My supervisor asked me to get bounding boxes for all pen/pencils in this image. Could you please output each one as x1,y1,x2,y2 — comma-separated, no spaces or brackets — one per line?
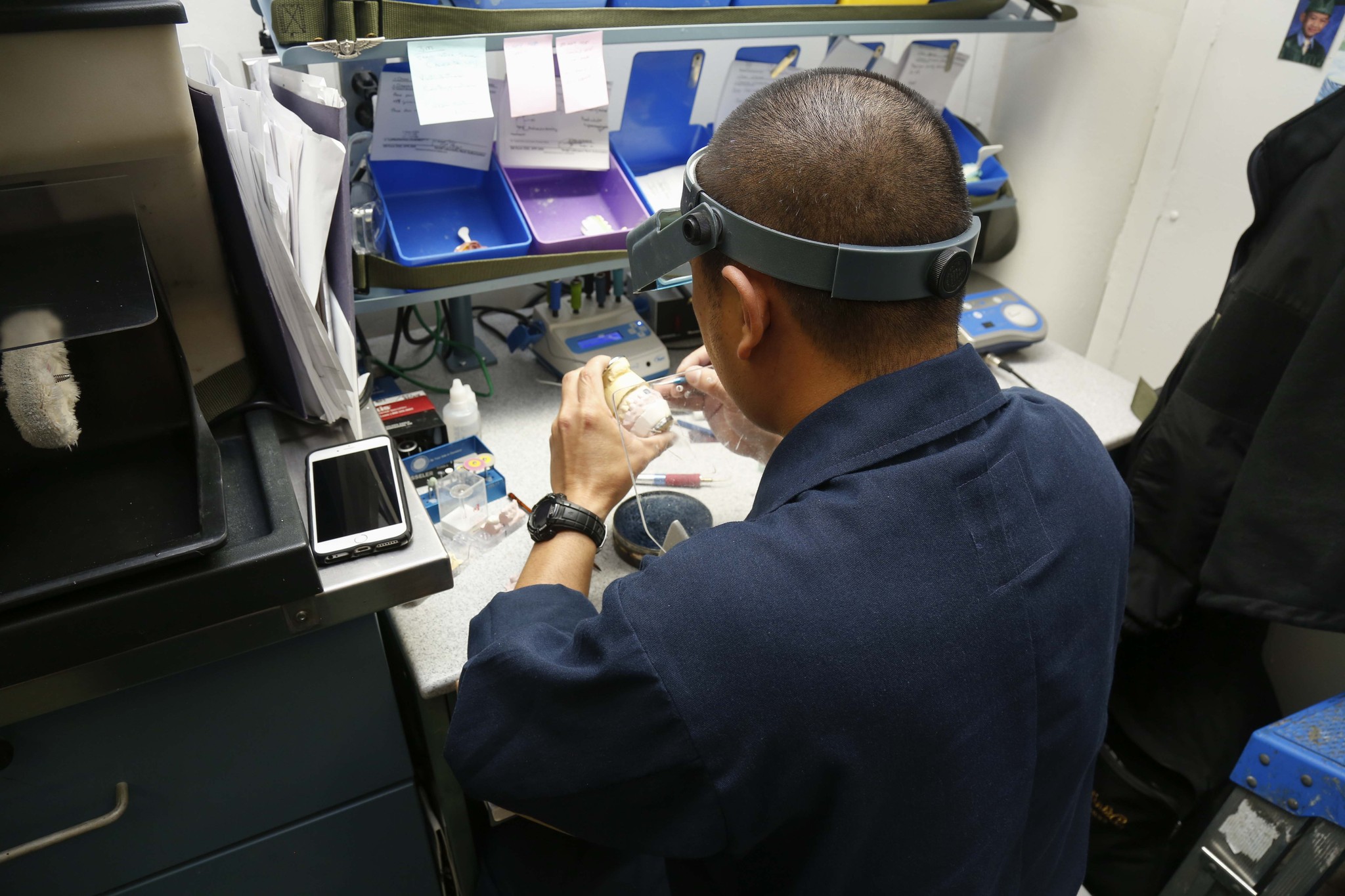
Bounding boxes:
509,494,603,573
674,417,714,437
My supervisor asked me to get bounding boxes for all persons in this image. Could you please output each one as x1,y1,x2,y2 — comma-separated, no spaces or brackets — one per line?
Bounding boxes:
1080,84,1345,896
444,66,1135,896
1279,0,1345,68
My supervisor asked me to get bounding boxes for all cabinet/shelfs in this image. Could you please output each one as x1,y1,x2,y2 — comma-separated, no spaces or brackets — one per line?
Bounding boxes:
255,0,1143,895
0,612,438,896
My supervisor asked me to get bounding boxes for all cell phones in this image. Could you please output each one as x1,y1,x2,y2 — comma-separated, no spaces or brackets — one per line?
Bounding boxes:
304,434,413,566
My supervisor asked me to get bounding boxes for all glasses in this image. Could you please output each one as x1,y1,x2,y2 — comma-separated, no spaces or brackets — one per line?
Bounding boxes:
625,203,723,296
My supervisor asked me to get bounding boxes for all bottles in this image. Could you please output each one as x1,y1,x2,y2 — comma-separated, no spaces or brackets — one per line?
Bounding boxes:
442,378,481,443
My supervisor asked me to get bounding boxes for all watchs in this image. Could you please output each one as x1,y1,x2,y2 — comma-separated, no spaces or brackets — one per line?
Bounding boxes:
527,494,607,554
